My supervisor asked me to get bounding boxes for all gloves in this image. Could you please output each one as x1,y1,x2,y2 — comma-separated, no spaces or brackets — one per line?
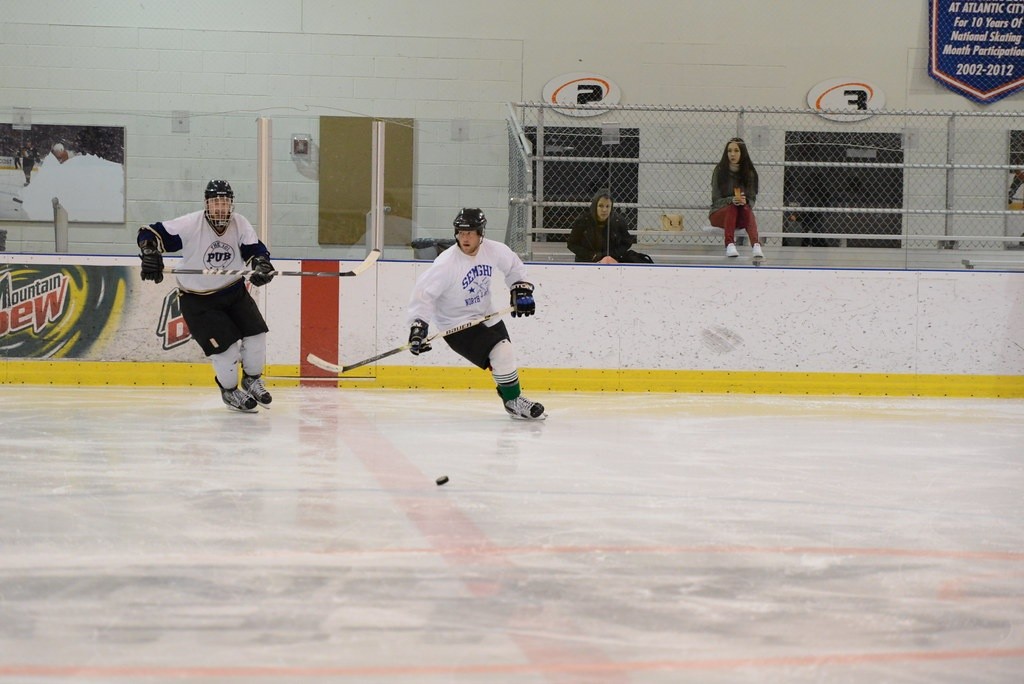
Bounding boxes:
249,256,275,287
138,246,165,284
510,281,535,318
408,318,432,355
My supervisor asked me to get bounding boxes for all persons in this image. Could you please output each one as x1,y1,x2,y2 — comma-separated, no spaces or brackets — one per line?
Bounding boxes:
708,137,764,257
408,208,548,420
9,146,21,170
23,142,43,186
136,179,275,414
51,142,68,164
567,188,632,264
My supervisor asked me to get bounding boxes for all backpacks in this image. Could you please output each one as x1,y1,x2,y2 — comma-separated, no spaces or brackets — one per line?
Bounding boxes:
620,250,654,264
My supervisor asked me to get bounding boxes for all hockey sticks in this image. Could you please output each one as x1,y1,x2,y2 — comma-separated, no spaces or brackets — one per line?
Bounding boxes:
162,248,382,277
304,306,515,374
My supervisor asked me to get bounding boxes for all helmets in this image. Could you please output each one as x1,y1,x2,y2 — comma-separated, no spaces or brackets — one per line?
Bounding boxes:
205,179,234,198
453,208,486,232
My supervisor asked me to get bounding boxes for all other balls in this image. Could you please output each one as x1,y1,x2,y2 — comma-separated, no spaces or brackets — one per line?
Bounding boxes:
435,475,450,486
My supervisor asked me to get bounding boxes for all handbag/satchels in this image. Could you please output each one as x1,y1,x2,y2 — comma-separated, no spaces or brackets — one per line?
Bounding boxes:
662,214,684,231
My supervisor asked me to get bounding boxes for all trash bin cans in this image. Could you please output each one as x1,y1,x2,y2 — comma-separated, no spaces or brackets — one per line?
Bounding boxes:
411,238,458,260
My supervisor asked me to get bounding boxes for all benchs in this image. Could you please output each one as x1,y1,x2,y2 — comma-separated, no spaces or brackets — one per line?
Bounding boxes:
530,228,1024,271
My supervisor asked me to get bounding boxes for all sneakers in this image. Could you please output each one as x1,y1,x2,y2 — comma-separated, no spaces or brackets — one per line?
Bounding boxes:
752,242,763,258
496,387,546,420
241,370,272,409
726,243,739,256
215,376,260,413
